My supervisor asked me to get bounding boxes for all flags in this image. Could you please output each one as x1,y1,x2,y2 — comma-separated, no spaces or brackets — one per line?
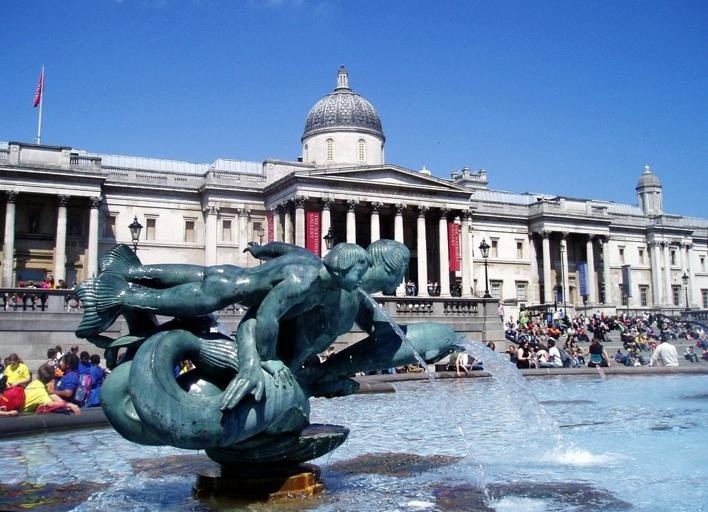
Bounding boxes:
32,68,43,109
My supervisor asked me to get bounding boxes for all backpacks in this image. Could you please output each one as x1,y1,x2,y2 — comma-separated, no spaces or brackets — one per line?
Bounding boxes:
73,374,92,402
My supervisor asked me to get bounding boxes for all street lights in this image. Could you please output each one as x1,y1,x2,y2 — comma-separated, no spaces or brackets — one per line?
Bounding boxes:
128,213,143,255
681,272,691,311
257,225,265,265
559,243,569,329
478,238,492,298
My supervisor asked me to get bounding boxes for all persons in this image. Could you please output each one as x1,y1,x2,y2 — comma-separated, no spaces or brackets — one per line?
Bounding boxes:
0,275,198,420
73,238,414,413
92,236,376,391
327,346,335,360
498,303,708,369
455,341,495,374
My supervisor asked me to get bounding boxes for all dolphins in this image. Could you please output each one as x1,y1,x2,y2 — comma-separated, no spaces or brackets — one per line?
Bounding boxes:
294,306,466,389
